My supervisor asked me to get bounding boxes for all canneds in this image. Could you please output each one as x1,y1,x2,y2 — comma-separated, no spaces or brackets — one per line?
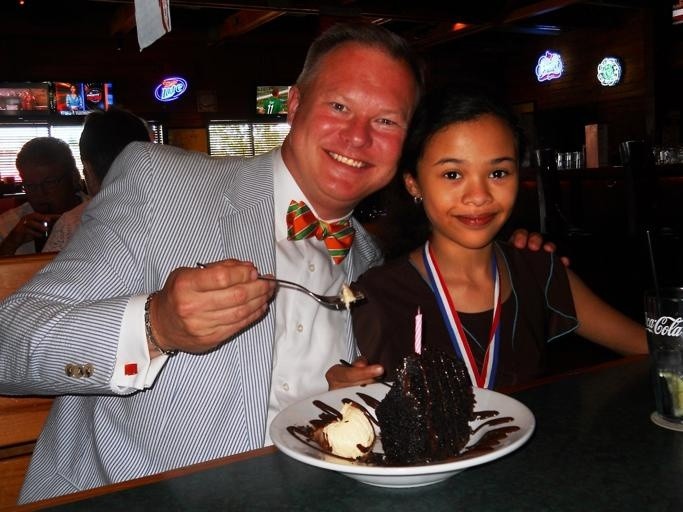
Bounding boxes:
553,151,582,172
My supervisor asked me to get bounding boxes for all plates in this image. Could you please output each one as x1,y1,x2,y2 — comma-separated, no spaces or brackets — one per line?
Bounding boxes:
265,378,536,489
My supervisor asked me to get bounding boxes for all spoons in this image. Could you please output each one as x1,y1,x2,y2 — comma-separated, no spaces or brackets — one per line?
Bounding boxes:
193,259,368,309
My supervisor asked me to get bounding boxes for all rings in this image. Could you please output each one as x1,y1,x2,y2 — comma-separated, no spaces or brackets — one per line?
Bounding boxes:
22,219,27,225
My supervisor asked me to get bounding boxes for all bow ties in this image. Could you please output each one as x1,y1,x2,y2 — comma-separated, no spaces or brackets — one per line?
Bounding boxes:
286,200,356,265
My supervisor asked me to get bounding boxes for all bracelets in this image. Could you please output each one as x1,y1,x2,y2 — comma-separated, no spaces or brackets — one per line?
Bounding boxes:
142,289,179,359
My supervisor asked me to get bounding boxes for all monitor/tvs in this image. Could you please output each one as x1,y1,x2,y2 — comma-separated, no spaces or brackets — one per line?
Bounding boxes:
53,80,115,120
256,85,293,115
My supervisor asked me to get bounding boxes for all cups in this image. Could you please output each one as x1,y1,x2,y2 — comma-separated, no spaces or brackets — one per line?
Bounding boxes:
640,286,683,423
32,204,54,254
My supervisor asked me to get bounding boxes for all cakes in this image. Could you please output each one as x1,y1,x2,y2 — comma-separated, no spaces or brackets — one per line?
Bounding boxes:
310,346,475,466
335,282,358,311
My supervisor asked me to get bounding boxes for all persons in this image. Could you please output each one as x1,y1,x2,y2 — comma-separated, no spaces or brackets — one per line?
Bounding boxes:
0,136,78,257
64,84,83,111
323,82,651,393
0,17,570,505
40,105,152,254
20,89,35,111
